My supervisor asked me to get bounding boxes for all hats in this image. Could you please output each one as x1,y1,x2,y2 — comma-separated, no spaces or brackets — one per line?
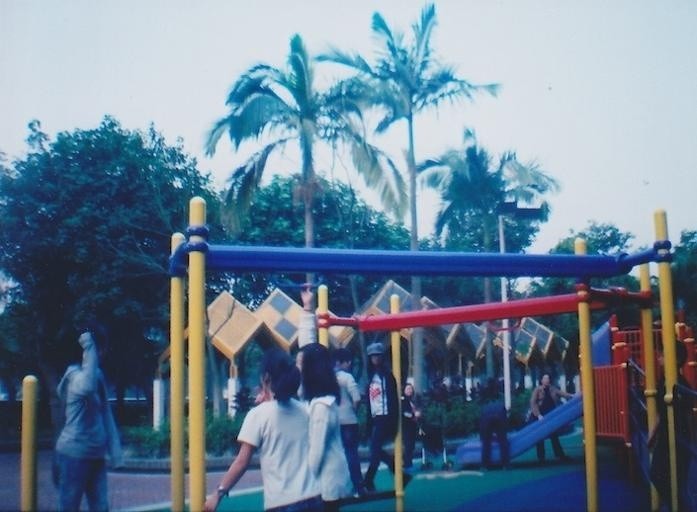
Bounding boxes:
367,342,385,356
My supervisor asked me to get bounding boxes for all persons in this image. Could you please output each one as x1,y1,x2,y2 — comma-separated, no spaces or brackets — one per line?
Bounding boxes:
478,398,517,471
643,337,692,508
201,346,326,512
253,343,354,512
364,341,413,493
47,324,127,512
528,372,575,467
398,381,424,469
334,347,369,500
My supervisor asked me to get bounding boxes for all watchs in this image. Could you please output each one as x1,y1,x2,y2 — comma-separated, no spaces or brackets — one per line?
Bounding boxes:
215,483,231,499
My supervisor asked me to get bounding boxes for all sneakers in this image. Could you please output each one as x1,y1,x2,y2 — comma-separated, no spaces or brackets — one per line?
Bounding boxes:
349,472,413,499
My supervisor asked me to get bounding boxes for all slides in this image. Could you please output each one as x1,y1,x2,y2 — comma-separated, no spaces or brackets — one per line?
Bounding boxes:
456,392,583,470
